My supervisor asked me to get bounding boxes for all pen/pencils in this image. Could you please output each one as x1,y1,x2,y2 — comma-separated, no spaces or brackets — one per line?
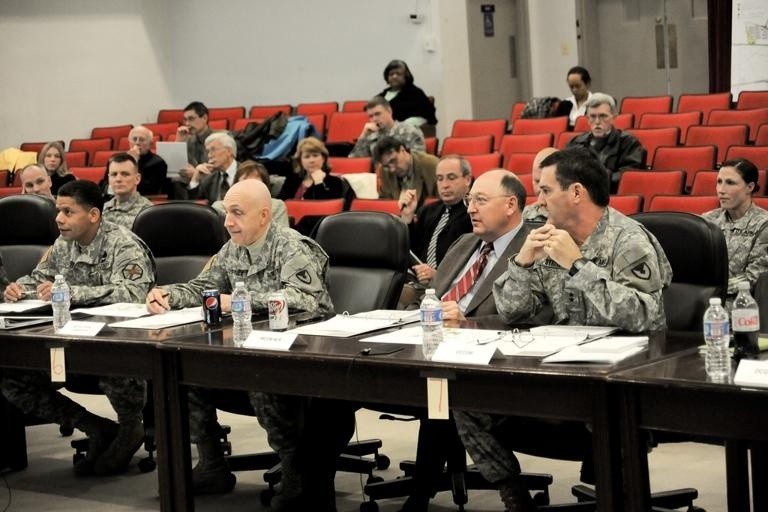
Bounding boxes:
150,293,169,303
22,291,37,295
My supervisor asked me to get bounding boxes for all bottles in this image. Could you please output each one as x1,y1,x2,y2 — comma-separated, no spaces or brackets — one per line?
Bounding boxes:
703,298,733,378
232,281,251,344
419,287,445,355
51,274,72,329
734,280,763,361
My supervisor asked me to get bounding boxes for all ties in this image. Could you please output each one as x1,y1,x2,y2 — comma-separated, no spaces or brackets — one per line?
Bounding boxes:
441,246,492,302
220,173,230,200
426,209,450,268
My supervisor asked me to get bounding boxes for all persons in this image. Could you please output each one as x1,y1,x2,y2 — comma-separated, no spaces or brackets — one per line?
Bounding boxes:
348,97,426,158
397,155,476,310
452,145,674,512
174,101,214,176
570,92,647,195
277,137,357,213
524,147,560,222
187,132,240,202
101,152,153,231
271,169,530,512
126,126,167,195
1,180,158,478
698,157,768,320
20,164,57,205
38,140,76,195
373,135,440,210
145,178,334,511
212,160,288,227
563,66,593,132
377,60,438,126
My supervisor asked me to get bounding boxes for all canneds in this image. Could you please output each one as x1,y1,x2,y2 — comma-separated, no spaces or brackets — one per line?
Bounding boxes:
267,292,290,329
201,289,223,325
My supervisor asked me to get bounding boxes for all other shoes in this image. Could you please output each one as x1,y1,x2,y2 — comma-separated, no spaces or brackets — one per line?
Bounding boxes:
271,471,304,510
191,469,235,496
499,475,534,512
278,492,337,512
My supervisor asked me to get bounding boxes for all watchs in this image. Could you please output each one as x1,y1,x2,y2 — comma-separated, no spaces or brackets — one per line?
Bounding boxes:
568,255,588,277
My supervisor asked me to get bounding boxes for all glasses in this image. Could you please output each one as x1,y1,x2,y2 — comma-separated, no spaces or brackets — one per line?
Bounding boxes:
462,192,508,208
476,328,534,348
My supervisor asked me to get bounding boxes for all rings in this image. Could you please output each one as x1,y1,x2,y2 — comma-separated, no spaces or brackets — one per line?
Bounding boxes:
422,272,425,276
546,242,549,247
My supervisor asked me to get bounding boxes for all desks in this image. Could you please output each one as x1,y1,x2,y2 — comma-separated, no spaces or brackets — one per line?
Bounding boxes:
0,298,765,510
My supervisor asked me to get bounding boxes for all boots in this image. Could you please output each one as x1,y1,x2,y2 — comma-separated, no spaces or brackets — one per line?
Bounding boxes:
74,414,120,476
94,409,146,476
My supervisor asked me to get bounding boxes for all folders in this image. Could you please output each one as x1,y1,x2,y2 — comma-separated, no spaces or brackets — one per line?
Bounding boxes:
0,315,53,329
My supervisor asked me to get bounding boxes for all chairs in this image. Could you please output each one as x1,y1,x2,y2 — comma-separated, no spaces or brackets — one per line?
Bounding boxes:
0,91,768,511
